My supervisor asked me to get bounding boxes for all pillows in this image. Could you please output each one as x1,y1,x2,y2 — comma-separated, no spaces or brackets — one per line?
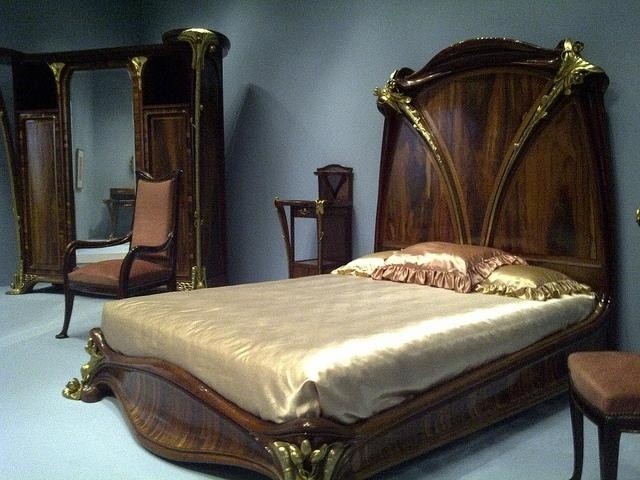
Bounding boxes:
331,241,591,301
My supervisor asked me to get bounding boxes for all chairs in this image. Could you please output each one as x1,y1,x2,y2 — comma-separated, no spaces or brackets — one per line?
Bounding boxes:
56,168,183,341
568,209,640,479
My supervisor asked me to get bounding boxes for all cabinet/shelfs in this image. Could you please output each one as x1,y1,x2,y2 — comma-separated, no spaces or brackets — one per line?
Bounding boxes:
0,27,231,294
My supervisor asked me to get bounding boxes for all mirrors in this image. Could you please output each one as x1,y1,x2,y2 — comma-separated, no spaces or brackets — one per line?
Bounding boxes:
65,68,137,269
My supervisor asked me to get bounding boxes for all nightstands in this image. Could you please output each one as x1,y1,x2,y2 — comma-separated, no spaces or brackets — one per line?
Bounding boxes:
274,165,351,278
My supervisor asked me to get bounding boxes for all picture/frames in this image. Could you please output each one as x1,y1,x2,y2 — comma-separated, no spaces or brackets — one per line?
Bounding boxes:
75,148,84,190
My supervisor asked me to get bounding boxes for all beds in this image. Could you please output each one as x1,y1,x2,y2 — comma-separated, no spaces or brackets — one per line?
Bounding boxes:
60,37,621,480
